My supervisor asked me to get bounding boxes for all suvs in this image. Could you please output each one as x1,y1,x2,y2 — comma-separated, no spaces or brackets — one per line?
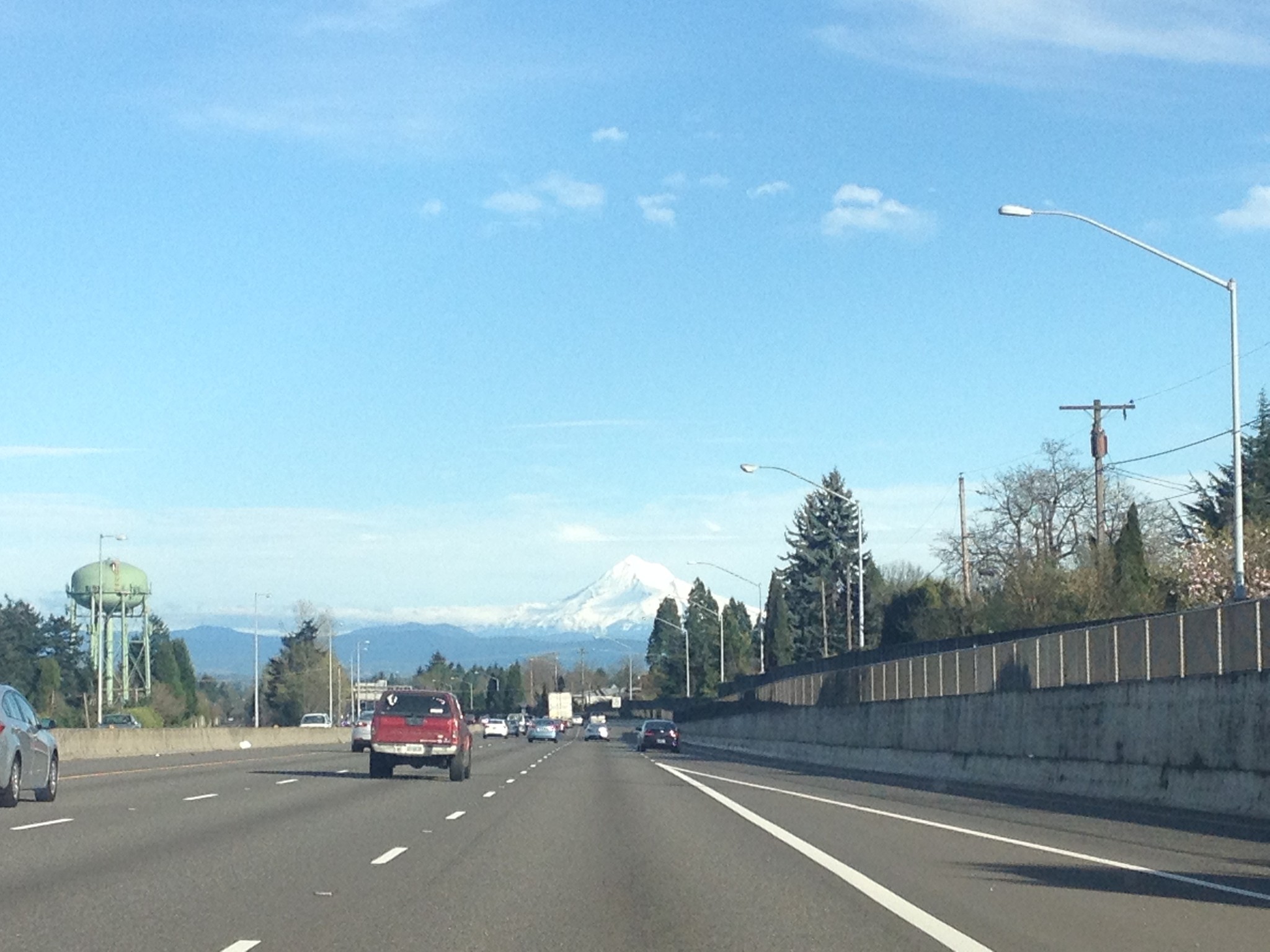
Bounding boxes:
368,687,474,782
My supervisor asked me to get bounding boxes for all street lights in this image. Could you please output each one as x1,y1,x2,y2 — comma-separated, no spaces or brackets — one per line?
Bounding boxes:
593,634,633,702
997,205,1250,603
641,616,690,698
739,463,866,648
680,597,724,683
328,621,344,726
450,676,473,710
356,638,370,726
432,679,453,693
254,590,273,729
687,560,766,675
96,531,127,726
474,670,499,691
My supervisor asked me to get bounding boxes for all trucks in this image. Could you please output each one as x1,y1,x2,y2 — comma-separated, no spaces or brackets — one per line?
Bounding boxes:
547,691,573,722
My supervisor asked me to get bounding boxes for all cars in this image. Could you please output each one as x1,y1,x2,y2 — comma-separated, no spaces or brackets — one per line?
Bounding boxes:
462,708,611,743
350,709,375,753
100,712,142,729
583,723,611,742
0,683,60,809
298,712,332,729
635,718,680,753
